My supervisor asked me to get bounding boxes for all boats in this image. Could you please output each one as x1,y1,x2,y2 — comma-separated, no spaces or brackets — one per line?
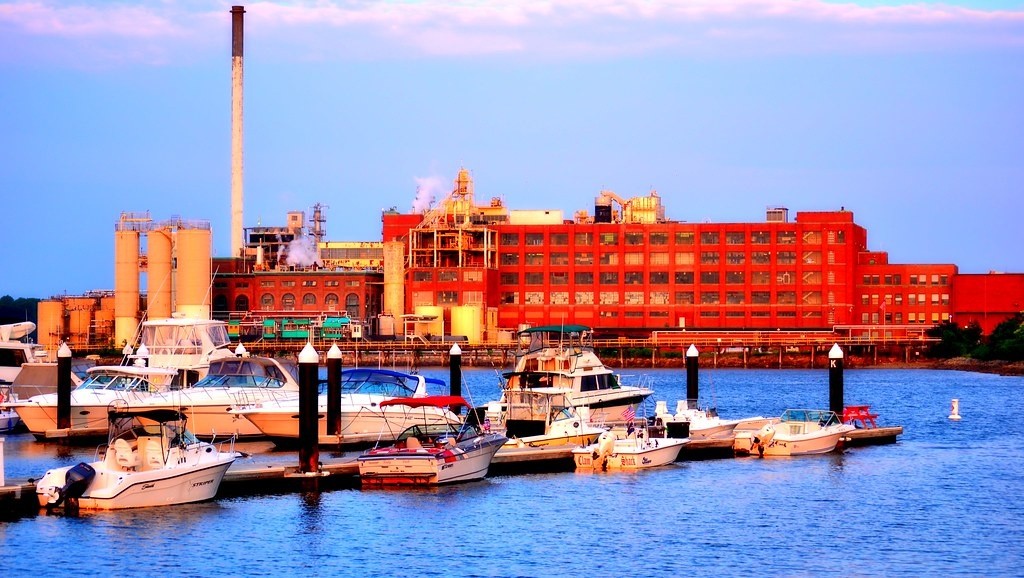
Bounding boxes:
732,409,857,456
356,397,509,487
674,399,763,430
0,366,179,441
502,406,605,449
571,417,691,469
481,325,654,437
228,367,460,448
35,409,243,510
114,353,299,439
0,385,20,432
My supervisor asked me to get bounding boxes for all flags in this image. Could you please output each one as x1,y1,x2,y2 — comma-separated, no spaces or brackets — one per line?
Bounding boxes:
621,405,635,422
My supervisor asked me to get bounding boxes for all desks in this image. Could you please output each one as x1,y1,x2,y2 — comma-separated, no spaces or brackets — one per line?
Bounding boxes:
843,406,877,430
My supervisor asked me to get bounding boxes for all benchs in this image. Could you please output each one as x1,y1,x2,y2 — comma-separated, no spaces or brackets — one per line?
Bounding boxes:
842,414,880,430
